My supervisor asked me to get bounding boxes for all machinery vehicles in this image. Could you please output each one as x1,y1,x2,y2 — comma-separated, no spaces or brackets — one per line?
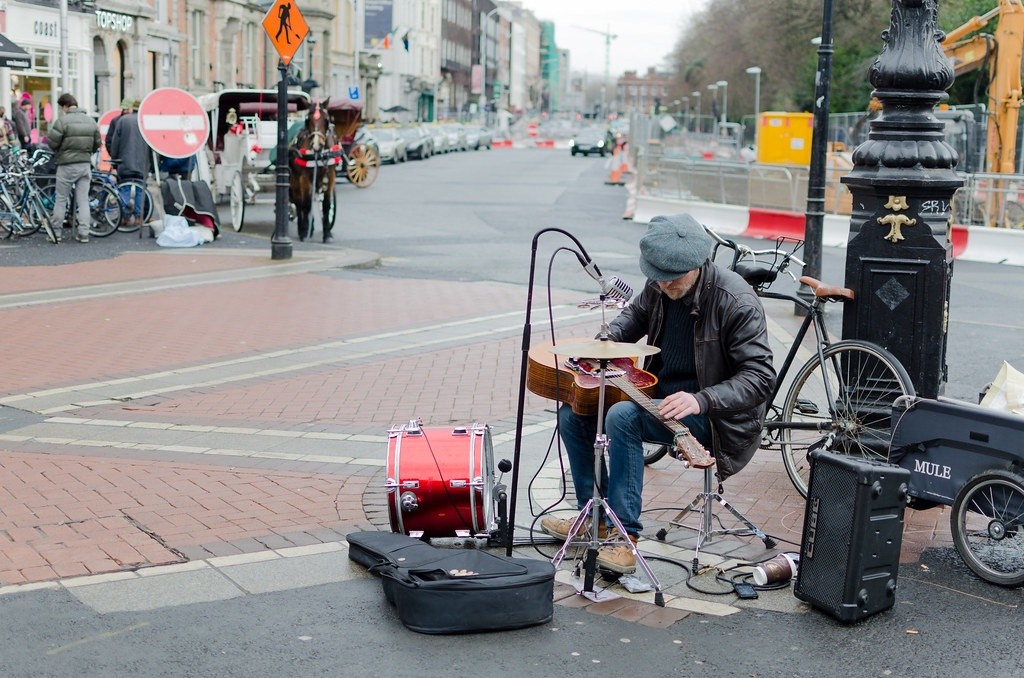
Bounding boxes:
749,1,1024,230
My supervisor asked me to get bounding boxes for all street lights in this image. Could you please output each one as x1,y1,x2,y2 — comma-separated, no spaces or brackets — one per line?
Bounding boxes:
706,84,720,122
674,100,683,126
717,81,729,122
681,96,690,129
745,65,762,147
691,91,702,133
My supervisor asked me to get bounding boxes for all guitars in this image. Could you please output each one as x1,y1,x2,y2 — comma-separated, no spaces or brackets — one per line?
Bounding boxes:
527,335,716,470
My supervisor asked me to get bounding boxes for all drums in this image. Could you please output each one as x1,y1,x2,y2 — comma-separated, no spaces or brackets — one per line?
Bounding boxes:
386,424,496,540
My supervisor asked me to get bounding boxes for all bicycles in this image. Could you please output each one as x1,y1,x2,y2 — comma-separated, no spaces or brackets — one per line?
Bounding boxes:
0,142,154,244
699,221,917,502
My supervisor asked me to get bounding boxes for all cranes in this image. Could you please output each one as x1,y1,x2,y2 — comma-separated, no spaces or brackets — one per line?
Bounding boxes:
572,23,618,76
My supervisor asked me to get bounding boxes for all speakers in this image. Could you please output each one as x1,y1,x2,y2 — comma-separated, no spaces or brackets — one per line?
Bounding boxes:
793,451,909,626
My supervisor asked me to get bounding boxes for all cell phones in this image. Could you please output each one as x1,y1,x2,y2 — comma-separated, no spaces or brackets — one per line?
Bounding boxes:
732,583,758,598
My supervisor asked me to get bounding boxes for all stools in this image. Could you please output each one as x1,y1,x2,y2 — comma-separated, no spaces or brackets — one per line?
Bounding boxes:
654,448,776,576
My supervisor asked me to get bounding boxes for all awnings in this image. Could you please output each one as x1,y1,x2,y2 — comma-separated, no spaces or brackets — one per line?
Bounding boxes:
0,34,31,68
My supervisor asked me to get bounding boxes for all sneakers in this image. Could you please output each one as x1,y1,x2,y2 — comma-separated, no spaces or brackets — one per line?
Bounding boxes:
595,527,637,574
541,514,607,542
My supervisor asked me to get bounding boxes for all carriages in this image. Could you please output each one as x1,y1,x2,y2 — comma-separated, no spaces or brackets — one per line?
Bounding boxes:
190,86,380,243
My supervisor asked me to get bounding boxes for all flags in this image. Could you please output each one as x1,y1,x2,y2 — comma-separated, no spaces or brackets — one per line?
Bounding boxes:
401,34,408,51
384,31,394,49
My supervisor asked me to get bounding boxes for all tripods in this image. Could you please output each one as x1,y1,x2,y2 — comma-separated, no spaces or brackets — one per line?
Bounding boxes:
551,360,666,608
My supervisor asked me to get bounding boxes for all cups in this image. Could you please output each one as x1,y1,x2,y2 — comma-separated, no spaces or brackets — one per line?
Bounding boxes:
753,553,796,586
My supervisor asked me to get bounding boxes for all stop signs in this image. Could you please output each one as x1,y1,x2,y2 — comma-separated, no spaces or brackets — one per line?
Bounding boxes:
96,109,124,153
526,124,541,138
137,87,211,159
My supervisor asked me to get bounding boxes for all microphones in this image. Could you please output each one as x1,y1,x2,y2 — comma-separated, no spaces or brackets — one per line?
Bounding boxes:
576,254,635,303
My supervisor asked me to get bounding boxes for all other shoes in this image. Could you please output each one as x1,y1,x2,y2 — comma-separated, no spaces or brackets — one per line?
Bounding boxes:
76,234,88,243
47,235,61,241
121,217,130,226
134,216,141,225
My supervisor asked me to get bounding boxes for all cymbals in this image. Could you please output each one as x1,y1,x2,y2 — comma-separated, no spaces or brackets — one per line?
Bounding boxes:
548,339,663,359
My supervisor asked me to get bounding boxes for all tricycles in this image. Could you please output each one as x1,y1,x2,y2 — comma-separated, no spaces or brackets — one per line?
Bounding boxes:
885,392,1024,589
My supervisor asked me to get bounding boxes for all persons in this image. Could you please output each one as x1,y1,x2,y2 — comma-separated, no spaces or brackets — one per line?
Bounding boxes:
541,214,777,573
111,98,150,225
15,100,31,154
46,94,102,243
159,155,196,181
0,106,15,173
104,98,134,170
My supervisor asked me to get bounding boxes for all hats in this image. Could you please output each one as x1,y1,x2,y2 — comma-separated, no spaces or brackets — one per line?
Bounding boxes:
132,99,142,111
639,212,712,282
120,98,134,110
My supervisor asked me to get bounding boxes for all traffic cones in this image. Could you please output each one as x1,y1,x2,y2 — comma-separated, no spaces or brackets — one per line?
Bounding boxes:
502,130,513,148
489,129,502,150
613,144,633,174
604,147,626,186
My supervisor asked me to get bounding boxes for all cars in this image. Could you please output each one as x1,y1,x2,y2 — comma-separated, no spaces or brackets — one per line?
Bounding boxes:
397,125,433,161
570,125,615,157
422,121,493,157
370,127,408,164
